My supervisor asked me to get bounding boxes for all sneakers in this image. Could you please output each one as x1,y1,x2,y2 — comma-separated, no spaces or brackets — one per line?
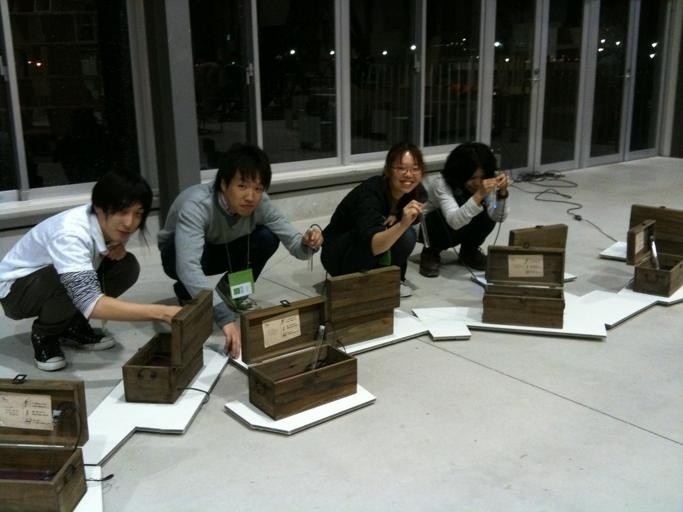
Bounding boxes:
29,318,66,371
399,281,412,298
62,321,115,351
174,282,195,306
216,286,262,313
419,244,440,277
457,245,488,270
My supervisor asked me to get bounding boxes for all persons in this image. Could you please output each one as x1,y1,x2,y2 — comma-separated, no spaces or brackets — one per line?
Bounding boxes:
319,140,430,298
411,141,510,278
156,143,324,358
1,170,184,372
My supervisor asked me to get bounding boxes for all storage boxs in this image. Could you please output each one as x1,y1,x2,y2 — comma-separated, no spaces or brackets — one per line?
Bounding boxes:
240,295,357,421
509,224,568,248
481,245,565,329
121,290,214,404
0,374,89,512
630,204,683,255
325,265,401,349
627,219,683,297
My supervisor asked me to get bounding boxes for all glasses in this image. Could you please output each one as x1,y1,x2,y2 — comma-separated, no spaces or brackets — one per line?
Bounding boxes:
388,165,422,176
